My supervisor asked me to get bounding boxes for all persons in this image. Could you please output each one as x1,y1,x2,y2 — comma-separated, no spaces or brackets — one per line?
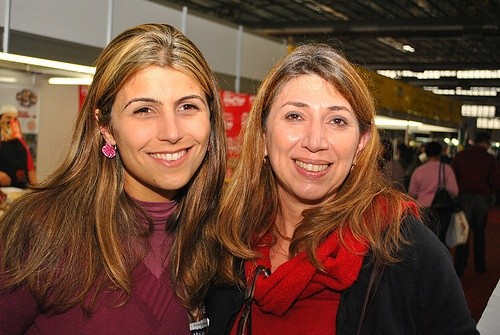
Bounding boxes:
453,133,498,275
206,44,480,335
380,139,500,209
409,141,458,243
0,22,232,335
0,107,36,188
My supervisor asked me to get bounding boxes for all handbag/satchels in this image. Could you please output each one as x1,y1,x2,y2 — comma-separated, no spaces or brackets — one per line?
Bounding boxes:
423,187,469,246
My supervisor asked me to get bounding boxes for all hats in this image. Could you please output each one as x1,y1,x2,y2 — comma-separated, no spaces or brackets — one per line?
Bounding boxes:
0,104,18,116
